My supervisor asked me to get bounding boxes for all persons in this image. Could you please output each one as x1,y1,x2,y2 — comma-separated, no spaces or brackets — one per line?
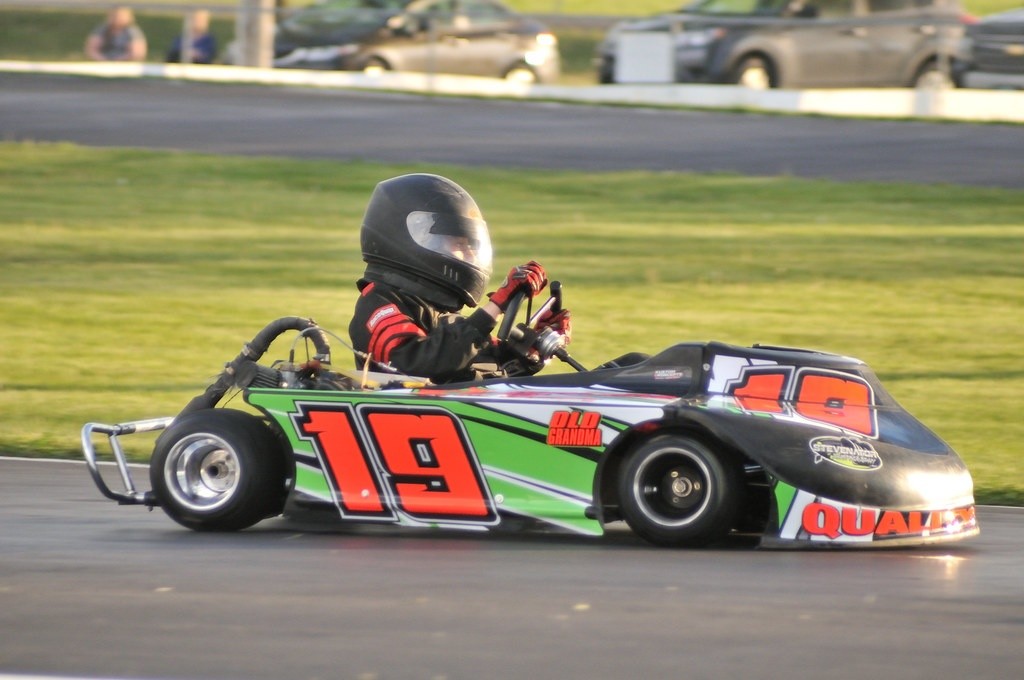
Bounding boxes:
77,2,151,65
347,174,571,383
166,0,223,65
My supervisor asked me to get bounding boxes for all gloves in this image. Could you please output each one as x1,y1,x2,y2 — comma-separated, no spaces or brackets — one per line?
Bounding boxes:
528,309,571,357
487,260,548,313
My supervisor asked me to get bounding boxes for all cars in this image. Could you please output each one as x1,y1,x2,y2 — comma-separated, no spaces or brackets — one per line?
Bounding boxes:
226,0,560,83
599,0,972,92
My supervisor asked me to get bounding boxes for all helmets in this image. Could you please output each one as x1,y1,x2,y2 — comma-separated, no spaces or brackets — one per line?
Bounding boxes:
360,173,494,312
